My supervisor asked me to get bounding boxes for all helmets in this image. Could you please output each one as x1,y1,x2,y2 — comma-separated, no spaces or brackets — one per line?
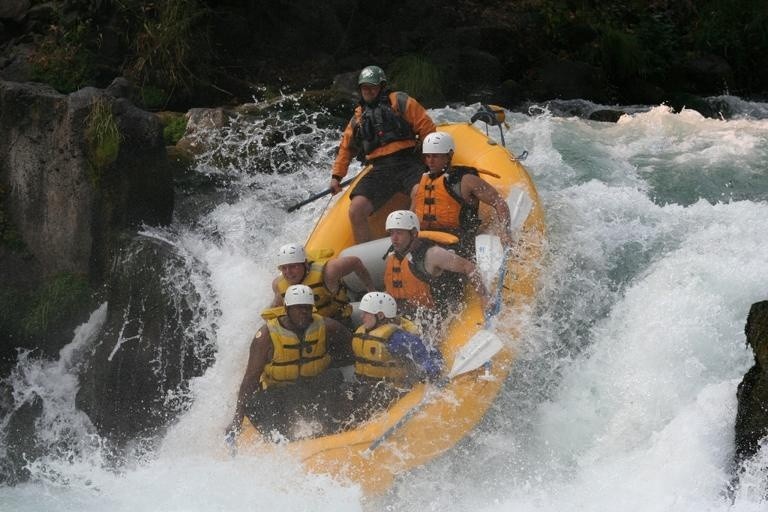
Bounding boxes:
358,65,387,87
359,291,397,319
422,131,455,155
277,242,306,267
283,284,315,308
385,209,421,234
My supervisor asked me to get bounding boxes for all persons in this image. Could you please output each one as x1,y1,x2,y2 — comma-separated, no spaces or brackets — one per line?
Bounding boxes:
268,240,377,329
221,285,350,459
379,206,492,340
409,130,511,254
329,63,436,247
347,289,442,423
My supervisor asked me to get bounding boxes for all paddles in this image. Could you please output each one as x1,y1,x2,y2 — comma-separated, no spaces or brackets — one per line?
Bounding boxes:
474,234,503,382
490,184,535,317
360,329,505,459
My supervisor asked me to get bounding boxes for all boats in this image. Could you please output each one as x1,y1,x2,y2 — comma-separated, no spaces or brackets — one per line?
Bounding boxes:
221,91,545,510
